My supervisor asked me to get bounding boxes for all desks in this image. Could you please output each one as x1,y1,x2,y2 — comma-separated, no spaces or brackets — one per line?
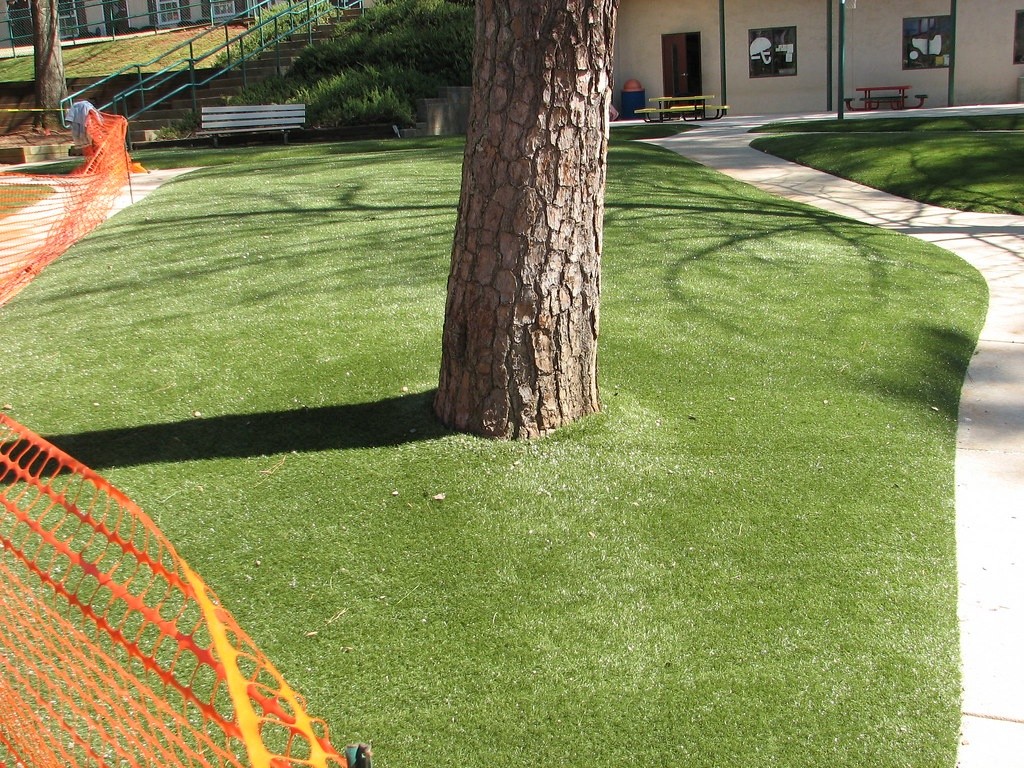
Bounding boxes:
855,85,912,111
648,95,719,123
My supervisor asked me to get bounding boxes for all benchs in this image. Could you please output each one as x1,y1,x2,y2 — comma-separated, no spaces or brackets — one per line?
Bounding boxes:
843,97,854,111
197,103,309,148
910,94,929,108
634,104,729,123
858,95,909,111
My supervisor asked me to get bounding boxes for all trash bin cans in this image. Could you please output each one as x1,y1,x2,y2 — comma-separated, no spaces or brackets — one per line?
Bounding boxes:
620,79,645,120
